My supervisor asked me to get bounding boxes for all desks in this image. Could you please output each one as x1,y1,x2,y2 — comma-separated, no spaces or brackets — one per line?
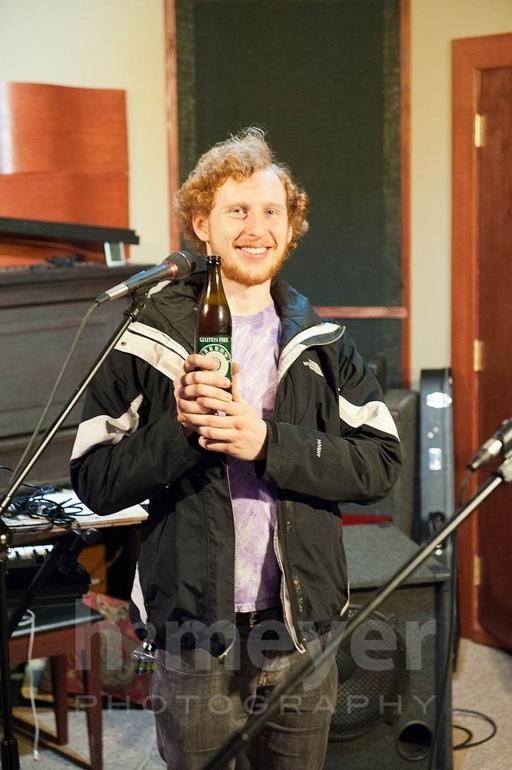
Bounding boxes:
1,487,148,770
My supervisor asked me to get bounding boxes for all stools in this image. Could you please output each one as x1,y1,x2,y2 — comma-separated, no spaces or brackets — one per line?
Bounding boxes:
1,601,109,769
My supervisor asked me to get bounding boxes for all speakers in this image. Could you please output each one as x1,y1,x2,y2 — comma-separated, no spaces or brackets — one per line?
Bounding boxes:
256,524,453,770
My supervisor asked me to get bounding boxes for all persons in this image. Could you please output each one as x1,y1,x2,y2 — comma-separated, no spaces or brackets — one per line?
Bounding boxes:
70,127,402,770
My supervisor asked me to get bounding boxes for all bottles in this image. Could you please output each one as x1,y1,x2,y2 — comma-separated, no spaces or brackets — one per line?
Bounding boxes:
194,254,234,416
130,641,153,672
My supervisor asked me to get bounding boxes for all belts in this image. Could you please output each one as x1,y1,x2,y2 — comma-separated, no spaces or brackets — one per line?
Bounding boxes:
235,604,285,631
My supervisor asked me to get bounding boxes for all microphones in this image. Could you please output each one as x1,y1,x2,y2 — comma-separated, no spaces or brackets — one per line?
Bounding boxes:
467,418,512,470
96,251,192,307
